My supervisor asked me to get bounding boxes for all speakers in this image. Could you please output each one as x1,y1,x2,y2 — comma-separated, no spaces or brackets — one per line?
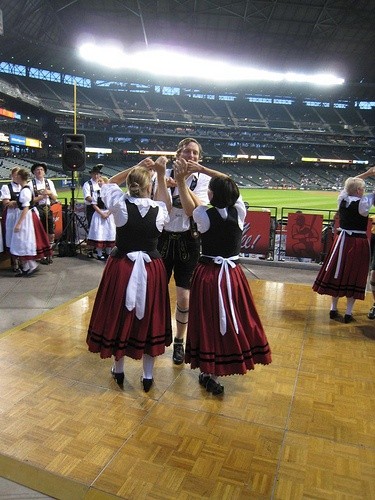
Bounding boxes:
62,134,86,172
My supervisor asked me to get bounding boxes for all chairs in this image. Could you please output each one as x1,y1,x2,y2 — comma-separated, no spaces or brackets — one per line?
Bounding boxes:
0,158,66,180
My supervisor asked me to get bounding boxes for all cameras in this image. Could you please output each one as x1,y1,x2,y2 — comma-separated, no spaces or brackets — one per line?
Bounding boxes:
173,195,182,208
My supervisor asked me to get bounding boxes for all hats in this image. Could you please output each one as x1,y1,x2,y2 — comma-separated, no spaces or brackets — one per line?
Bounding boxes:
31,163,47,174
89,166,103,174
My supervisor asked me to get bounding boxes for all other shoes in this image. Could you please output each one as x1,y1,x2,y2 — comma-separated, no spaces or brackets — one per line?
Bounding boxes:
26,266,40,276
87,250,92,258
15,268,30,277
40,258,48,265
46,258,52,263
12,267,21,272
98,253,110,260
368,303,375,319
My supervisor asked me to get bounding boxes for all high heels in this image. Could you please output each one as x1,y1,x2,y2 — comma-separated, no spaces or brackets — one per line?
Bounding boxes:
207,380,224,394
143,376,154,392
330,310,338,319
344,314,352,323
111,366,125,385
199,373,210,387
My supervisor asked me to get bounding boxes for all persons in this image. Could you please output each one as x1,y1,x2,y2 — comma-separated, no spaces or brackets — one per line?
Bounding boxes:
82,164,105,254
28,163,58,265
10,168,39,275
0,166,24,271
238,202,249,257
150,135,213,364
295,211,314,262
86,158,173,393
299,163,375,190
312,167,375,323
260,172,286,183
172,157,272,394
87,175,116,259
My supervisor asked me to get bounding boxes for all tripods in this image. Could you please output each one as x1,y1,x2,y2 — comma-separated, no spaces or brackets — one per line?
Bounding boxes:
52,171,88,256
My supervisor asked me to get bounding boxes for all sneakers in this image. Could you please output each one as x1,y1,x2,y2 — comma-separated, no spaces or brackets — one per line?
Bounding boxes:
173,343,185,364
